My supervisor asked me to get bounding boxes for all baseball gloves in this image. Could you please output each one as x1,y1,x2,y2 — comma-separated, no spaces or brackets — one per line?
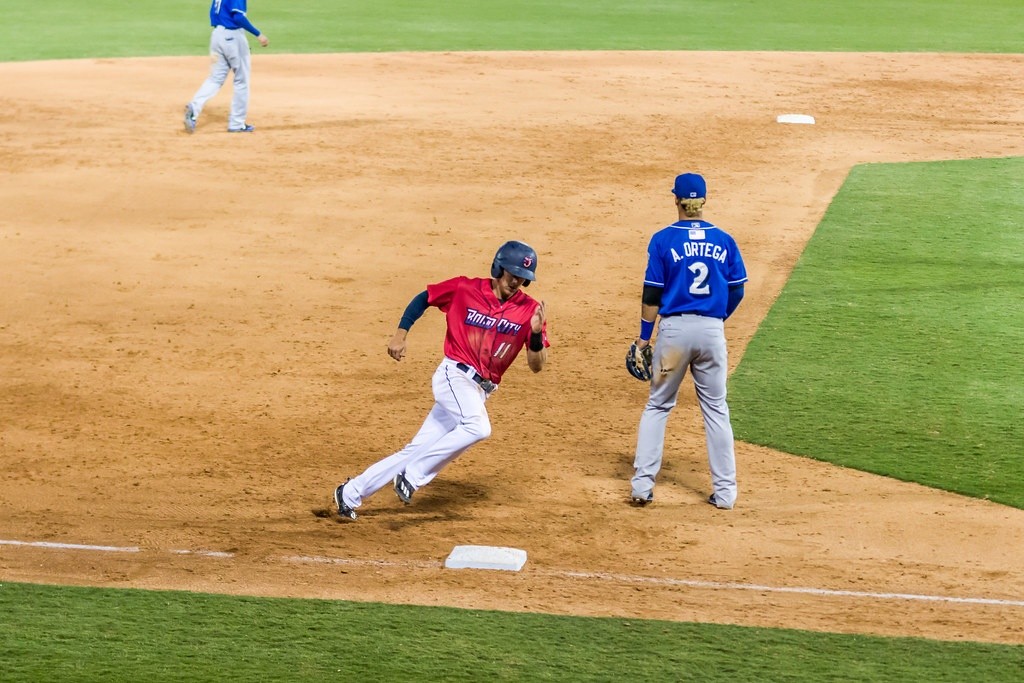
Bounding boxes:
625,342,653,382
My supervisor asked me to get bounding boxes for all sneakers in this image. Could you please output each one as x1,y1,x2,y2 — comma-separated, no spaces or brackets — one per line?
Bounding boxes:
708,493,717,505
183,103,196,132
393,470,415,505
334,477,356,520
228,124,254,132
632,490,653,503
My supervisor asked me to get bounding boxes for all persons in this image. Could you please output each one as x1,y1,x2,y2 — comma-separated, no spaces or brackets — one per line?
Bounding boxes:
333,240,550,520
626,172,748,511
182,0,269,133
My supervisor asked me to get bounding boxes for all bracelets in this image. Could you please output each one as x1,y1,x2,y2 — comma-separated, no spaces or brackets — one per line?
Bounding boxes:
529,329,543,352
640,317,656,341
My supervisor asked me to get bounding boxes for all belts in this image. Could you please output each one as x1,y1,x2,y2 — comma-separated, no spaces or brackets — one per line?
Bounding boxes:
457,363,494,394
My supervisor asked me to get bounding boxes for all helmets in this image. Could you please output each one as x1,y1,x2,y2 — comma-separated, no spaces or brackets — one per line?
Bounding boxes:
491,241,537,287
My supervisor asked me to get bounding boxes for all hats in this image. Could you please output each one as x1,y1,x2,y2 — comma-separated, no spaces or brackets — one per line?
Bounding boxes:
671,173,706,199
